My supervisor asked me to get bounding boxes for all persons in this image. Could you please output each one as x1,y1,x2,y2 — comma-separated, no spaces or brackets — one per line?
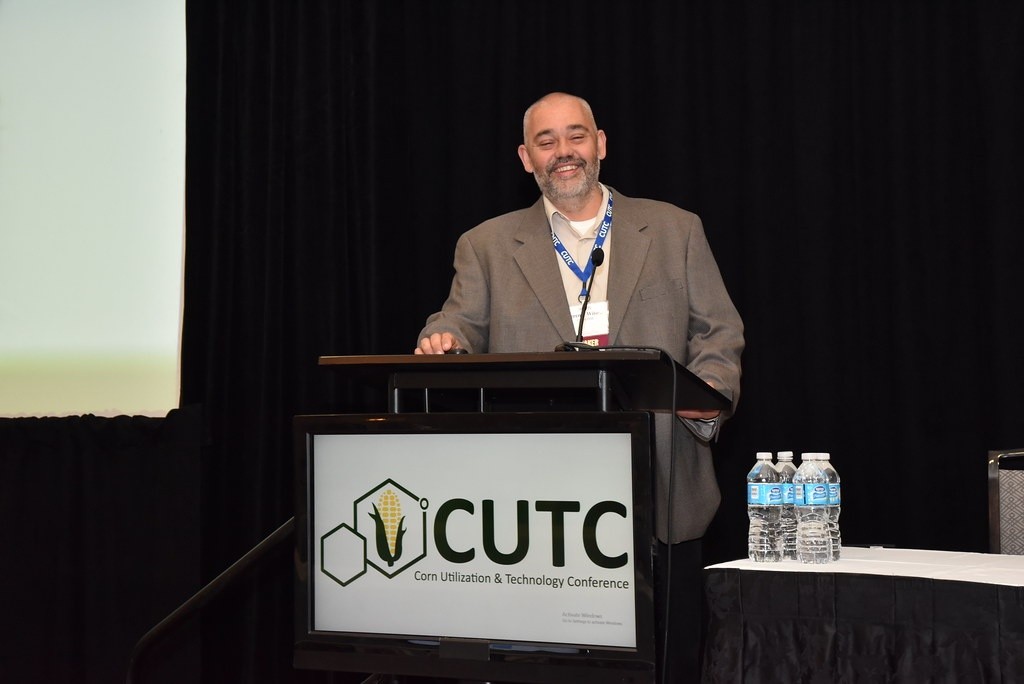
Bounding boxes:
414,92,746,684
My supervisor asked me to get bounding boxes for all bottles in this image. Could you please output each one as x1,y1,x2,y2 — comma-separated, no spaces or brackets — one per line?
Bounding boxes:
793,453,834,565
746,451,781,563
816,454,841,562
773,451,801,561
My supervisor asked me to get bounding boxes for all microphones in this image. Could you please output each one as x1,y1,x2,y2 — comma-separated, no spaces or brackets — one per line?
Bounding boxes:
555,248,604,353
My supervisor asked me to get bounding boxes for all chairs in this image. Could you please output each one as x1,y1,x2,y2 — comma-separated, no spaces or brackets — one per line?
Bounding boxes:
988,448,1024,555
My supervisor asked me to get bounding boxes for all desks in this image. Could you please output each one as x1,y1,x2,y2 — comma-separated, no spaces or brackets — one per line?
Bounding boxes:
702,546,1024,684
